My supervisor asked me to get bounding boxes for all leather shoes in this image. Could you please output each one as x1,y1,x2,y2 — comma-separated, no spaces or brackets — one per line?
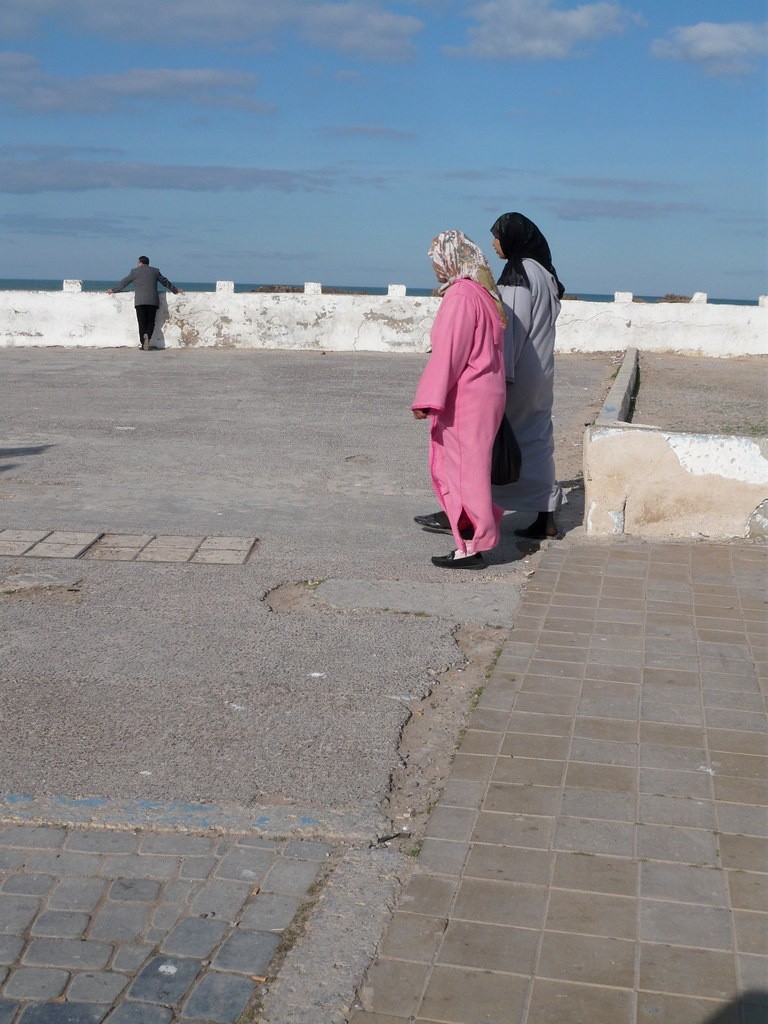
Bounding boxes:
431,550,488,570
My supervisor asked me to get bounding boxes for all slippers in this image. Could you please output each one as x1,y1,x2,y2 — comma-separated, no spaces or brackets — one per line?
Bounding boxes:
515,520,561,541
414,510,474,539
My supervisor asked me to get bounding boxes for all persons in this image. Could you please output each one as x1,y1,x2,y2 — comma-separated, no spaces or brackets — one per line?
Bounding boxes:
106,256,183,350
414,213,569,537
412,231,507,570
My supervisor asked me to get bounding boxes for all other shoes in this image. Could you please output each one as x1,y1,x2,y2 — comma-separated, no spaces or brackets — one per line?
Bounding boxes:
139,334,149,350
